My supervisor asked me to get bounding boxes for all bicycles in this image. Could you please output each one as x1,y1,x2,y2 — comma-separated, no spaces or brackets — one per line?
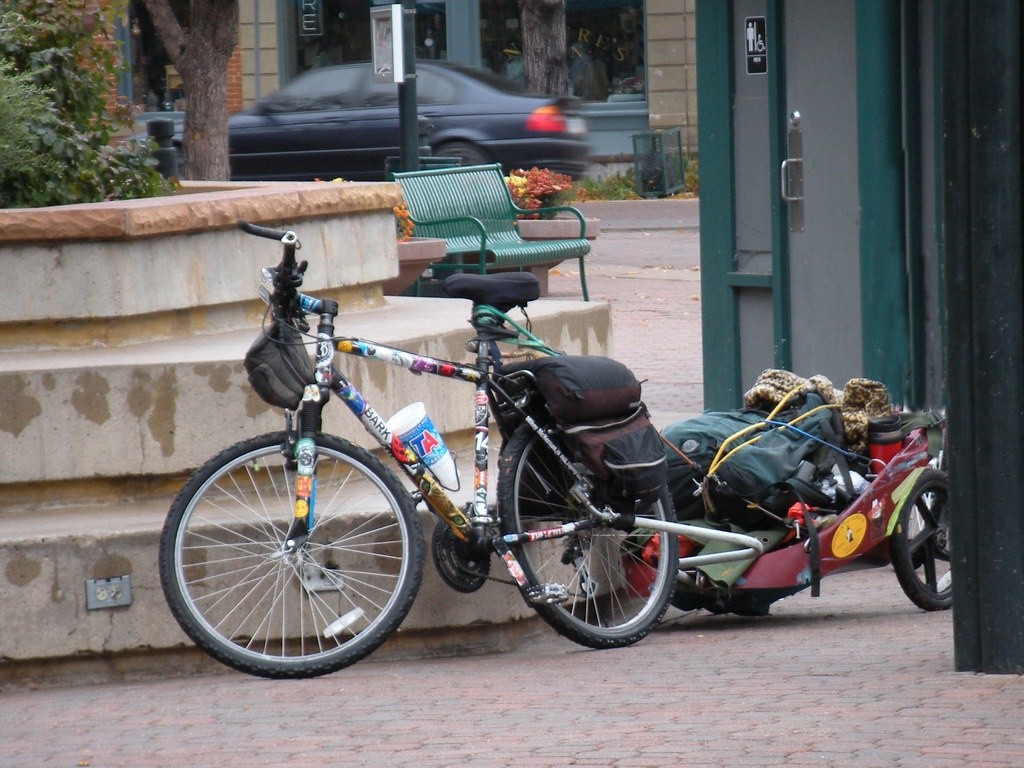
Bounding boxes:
157,220,679,679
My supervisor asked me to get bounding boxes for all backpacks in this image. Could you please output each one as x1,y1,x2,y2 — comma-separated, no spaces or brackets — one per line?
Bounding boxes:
658,390,846,532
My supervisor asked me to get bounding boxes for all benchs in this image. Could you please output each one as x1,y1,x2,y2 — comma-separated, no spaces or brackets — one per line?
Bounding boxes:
388,161,593,304
385,156,462,182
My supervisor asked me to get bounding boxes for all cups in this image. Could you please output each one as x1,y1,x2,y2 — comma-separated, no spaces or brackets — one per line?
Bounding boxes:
385,401,460,488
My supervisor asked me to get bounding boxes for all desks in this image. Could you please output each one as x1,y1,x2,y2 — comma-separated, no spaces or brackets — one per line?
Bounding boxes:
164,65,183,92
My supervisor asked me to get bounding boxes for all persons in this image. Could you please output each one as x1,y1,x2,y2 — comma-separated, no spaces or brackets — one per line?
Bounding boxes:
503,42,523,81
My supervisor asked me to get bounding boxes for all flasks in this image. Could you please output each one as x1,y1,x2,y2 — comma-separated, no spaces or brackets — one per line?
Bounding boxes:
867,414,905,477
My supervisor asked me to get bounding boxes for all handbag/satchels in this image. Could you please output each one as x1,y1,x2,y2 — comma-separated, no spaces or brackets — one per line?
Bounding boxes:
491,355,667,521
244,320,317,411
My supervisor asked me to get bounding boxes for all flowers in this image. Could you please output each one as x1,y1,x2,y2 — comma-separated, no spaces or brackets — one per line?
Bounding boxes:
613,76,644,94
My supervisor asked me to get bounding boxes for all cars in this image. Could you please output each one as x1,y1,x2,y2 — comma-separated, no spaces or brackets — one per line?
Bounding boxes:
172,60,593,181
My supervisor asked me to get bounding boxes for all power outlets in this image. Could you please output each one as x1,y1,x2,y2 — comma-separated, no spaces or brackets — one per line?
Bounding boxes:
85,575,133,610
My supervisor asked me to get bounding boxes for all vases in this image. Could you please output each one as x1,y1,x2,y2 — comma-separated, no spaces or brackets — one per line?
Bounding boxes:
607,94,644,101
